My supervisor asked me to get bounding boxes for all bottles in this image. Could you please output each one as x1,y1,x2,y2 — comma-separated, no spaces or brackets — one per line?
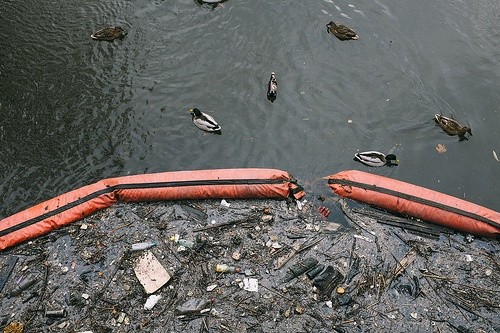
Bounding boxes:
174,296,215,317
130,242,156,251
216,264,241,274
10,272,37,296
179,239,196,248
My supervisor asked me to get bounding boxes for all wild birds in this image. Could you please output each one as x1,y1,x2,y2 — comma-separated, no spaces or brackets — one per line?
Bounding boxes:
91,27,126,41
267,71,278,97
188,108,221,132
433,114,473,137
327,21,360,41
354,150,399,167
